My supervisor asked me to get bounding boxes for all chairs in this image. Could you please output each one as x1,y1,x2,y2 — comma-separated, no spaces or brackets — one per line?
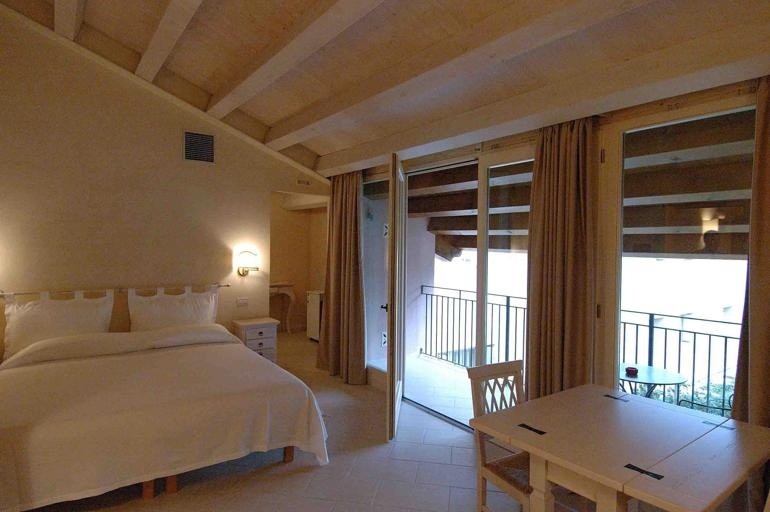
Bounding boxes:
468,357,532,511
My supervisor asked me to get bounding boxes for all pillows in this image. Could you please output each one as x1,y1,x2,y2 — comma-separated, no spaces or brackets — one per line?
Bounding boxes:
3,288,217,358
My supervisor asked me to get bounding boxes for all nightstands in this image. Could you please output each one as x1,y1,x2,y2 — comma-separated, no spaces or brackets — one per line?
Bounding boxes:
232,317,282,363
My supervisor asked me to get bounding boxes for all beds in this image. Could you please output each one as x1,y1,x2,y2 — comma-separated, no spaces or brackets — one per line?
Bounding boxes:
1,284,311,511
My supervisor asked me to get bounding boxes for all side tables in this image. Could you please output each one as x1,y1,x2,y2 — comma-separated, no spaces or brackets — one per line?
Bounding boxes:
617,363,686,399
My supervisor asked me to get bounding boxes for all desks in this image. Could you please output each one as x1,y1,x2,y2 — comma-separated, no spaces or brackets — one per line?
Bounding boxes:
469,383,770,511
